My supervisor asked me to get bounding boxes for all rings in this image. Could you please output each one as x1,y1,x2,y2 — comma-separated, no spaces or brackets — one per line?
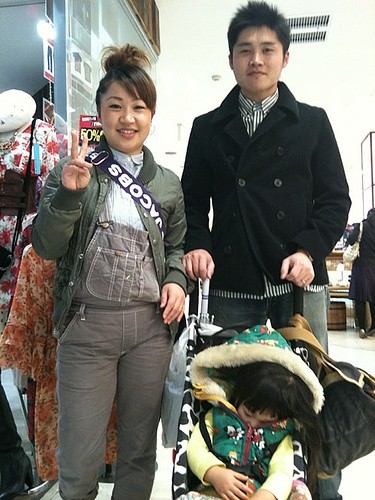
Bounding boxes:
301,279,306,284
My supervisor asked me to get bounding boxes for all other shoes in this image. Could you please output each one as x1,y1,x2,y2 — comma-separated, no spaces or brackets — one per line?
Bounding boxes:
366,327,375,335
359,329,367,338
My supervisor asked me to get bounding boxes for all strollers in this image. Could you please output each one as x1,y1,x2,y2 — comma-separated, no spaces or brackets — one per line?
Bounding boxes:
171,275,344,500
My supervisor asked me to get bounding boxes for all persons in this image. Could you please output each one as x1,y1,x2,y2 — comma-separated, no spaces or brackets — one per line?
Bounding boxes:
187,324,326,500
345,208,375,338
180,0,351,356
28,45,191,500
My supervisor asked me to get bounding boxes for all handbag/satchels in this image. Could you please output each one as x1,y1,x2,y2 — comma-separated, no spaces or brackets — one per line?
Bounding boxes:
274,312,375,479
343,222,364,264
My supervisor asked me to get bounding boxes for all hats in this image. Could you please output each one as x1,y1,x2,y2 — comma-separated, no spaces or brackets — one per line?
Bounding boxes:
0,89,36,133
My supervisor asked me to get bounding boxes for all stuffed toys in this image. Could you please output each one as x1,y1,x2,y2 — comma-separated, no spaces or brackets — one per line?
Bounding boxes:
0,89,60,174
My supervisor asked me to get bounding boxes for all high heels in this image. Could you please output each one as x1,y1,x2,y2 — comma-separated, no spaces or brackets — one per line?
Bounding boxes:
0,447,34,500
